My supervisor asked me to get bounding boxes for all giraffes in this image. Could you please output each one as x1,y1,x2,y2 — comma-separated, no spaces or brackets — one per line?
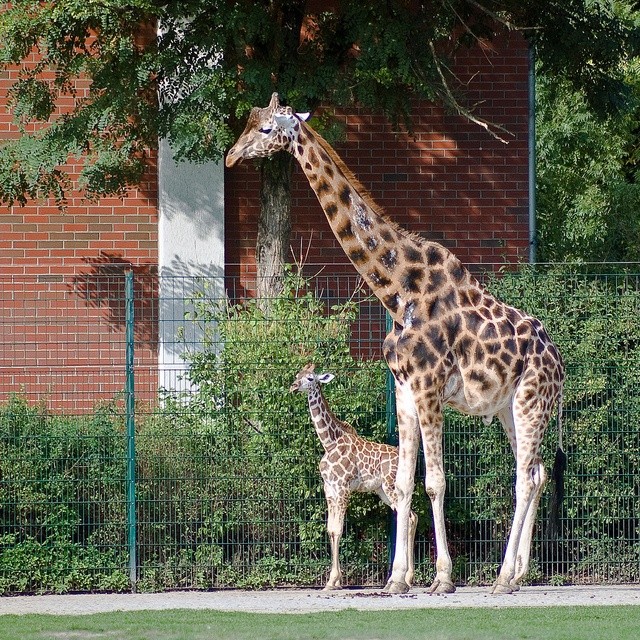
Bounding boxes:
226,92,568,593
291,363,416,589
